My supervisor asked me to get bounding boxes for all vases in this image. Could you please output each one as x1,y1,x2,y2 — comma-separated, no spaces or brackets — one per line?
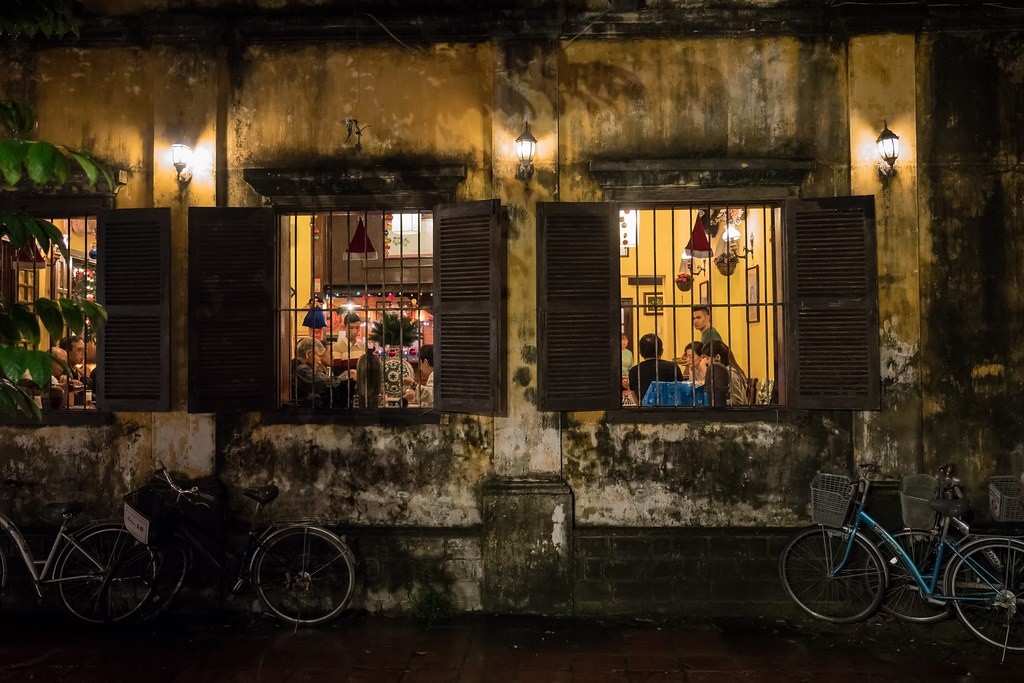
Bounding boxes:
716,262,736,276
676,281,693,291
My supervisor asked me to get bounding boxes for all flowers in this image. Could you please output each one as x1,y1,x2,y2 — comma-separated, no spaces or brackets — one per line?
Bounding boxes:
674,273,695,282
713,252,740,263
698,208,744,224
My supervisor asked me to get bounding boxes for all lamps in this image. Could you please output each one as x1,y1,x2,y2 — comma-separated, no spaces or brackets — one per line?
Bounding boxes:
514,119,535,178
682,249,706,277
340,298,361,310
392,213,421,235
723,223,753,259
873,115,903,178
168,137,194,186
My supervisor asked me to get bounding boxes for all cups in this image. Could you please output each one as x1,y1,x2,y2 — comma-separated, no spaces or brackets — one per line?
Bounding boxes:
406,389,415,403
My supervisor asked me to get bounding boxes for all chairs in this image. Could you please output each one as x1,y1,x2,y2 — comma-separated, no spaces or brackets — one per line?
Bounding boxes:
623,370,775,406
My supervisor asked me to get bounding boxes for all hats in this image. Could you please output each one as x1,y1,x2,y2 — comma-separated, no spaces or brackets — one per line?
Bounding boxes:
343,216,379,261
684,210,714,258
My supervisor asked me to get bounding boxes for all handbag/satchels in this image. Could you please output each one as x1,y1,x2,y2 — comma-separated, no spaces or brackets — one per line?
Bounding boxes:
12,235,47,269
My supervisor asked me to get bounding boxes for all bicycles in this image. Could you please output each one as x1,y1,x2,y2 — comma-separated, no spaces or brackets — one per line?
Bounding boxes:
863,462,1024,625
0,458,158,631
99,459,363,637
778,459,1024,665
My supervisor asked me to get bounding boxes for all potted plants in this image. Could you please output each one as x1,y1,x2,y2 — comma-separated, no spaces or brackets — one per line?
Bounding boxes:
368,312,424,407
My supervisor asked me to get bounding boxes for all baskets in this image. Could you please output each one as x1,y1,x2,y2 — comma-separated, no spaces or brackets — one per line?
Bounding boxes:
810,473,854,529
899,474,939,530
988,475,1024,522
122,484,173,546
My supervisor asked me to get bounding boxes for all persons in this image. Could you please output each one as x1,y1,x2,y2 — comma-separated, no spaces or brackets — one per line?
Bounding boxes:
330,313,365,353
682,341,748,406
629,333,683,402
291,336,387,407
691,307,721,345
19,335,97,409
621,334,633,376
404,343,434,406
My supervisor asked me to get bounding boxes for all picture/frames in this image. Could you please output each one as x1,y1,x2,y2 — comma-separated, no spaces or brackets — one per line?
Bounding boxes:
643,291,664,315
699,280,708,311
620,247,629,257
746,265,760,324
